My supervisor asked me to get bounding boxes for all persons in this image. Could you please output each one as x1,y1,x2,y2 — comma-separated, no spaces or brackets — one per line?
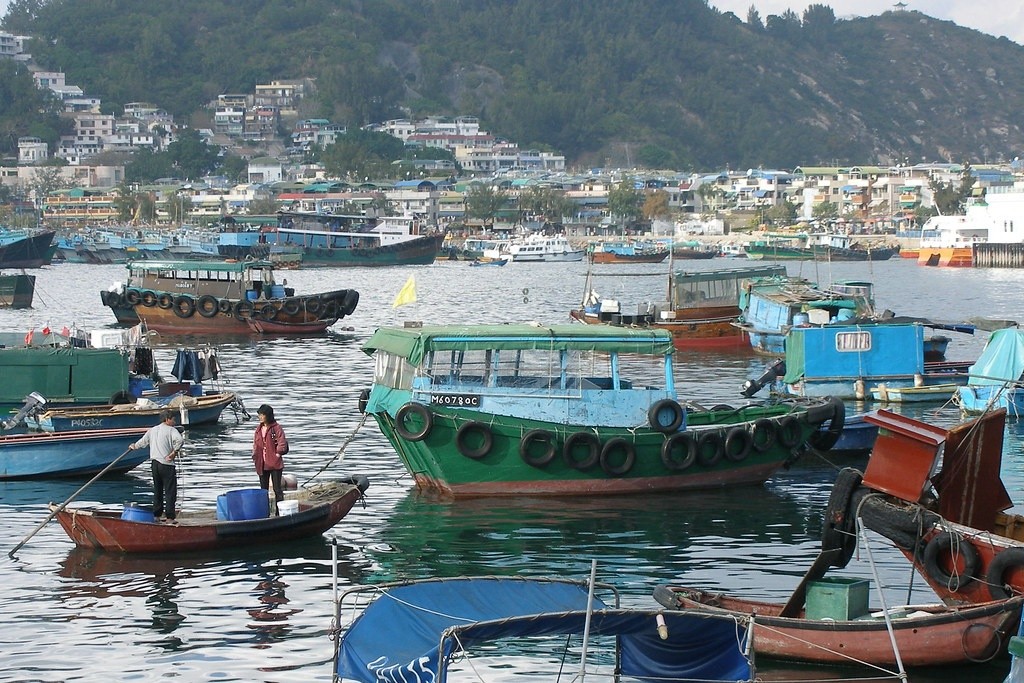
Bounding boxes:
129,410,184,524
252,404,287,516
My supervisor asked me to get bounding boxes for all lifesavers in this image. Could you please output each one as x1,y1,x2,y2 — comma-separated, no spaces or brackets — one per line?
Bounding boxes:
463,250,469,256
746,246,882,257
924,531,978,589
777,416,802,448
811,396,846,451
218,244,270,260
748,418,776,452
725,427,753,462
614,252,714,260
513,251,568,258
563,431,600,470
520,429,557,467
822,466,863,568
650,399,684,433
599,437,635,475
126,289,360,326
273,260,301,267
455,421,493,459
986,546,1024,601
697,432,725,466
661,432,696,471
358,389,371,414
108,389,136,411
395,401,433,442
305,236,435,258
106,291,132,309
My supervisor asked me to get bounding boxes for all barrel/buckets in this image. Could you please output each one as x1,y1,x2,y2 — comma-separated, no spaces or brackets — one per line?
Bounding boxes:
120,506,154,523
277,500,299,517
190,384,202,397
226,489,269,520
271,285,284,298
245,289,258,300
130,378,154,399
216,494,228,521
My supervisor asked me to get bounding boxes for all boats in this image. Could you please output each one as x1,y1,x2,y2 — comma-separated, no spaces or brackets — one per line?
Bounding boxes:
500,236,587,262
214,210,446,269
51,472,369,552
815,405,1023,664
649,573,1024,673
462,236,511,262
569,222,976,453
0,271,37,309
870,382,960,404
97,280,298,327
357,325,845,497
471,258,509,267
0,227,60,270
0,425,185,481
124,260,359,338
956,322,1024,421
0,316,236,434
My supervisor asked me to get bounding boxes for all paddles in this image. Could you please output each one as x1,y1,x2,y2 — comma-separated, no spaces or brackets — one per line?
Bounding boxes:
9,448,132,555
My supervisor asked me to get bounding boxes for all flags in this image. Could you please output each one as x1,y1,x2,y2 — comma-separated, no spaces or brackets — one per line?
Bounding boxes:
25,327,34,345
42,326,49,334
61,327,70,337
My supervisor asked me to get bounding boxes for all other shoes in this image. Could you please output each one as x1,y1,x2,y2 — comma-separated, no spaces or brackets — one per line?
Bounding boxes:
166,518,179,524
154,517,160,522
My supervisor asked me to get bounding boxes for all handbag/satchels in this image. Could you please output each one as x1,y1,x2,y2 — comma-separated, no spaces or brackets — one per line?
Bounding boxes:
271,427,289,455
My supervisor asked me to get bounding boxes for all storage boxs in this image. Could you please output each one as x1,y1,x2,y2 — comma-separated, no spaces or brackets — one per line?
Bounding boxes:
809,309,829,324
129,377,154,399
272,285,294,297
159,382,202,398
90,329,123,347
803,575,870,621
582,289,676,327
